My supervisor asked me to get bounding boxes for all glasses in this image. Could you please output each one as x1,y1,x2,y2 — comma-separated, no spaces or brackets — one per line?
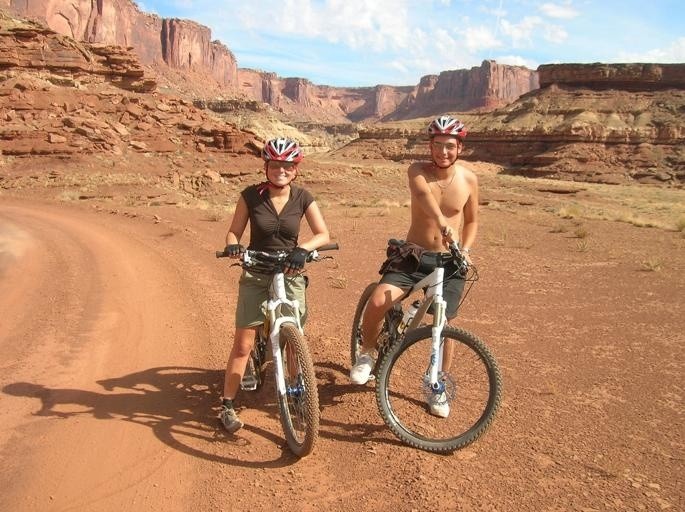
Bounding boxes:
267,161,296,171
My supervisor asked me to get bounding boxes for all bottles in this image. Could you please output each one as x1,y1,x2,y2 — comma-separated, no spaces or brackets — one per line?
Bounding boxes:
396,300,421,335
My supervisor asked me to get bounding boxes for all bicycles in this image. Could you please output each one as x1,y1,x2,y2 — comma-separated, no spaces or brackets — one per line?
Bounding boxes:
344,224,504,454
214,238,339,458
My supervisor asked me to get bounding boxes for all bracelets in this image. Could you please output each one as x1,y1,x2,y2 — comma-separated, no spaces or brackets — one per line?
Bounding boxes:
461,247,470,254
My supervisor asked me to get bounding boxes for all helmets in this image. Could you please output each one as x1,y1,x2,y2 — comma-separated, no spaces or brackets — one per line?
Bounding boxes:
262,137,303,163
428,115,467,139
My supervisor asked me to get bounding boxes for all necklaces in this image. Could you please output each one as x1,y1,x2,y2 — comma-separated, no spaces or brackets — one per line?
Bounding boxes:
432,164,457,195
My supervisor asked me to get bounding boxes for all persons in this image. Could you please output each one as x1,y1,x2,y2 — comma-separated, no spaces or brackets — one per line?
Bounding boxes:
216,136,331,436
347,116,480,418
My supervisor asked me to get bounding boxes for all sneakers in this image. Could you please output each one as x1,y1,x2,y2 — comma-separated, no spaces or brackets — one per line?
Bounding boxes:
295,396,322,420
429,389,450,419
218,405,244,434
350,352,374,386
